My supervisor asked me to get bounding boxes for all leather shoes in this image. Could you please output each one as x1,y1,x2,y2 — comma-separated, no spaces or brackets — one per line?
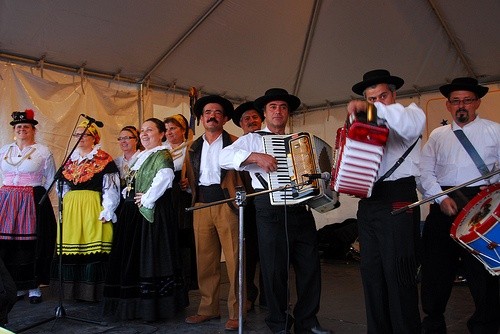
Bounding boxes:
225,319,239,330
294,321,334,334
185,313,221,324
275,330,290,334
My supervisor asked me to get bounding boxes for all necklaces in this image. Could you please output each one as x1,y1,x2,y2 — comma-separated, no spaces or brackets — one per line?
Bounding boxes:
127,150,154,192
4,146,36,166
73,159,89,185
122,158,138,186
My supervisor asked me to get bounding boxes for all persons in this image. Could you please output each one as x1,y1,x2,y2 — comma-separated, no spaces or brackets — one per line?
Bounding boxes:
0,265,26,334
112,125,148,322
121,118,190,322
226,100,266,333
415,76,500,334
163,114,199,321
219,87,334,334
185,94,255,330
0,110,56,303
55,120,121,327
347,69,426,334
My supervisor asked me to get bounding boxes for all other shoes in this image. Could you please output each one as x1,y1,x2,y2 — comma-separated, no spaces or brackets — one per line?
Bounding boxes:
29,288,43,305
247,300,253,311
16,291,27,300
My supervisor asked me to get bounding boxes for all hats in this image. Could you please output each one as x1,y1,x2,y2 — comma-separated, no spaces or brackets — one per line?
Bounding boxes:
254,88,301,118
193,95,234,121
439,77,489,99
232,101,265,127
10,109,38,125
352,69,404,96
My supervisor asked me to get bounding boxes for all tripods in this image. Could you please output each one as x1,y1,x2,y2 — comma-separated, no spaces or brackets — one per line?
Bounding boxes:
14,121,108,334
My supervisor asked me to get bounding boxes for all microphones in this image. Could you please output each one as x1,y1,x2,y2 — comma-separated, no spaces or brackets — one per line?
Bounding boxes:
81,114,104,128
303,171,330,182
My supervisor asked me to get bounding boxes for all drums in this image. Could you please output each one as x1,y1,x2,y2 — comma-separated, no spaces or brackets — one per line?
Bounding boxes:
450,182,500,278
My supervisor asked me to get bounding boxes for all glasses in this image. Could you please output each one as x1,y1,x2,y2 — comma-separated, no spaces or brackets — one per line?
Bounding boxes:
118,136,136,141
76,133,94,137
